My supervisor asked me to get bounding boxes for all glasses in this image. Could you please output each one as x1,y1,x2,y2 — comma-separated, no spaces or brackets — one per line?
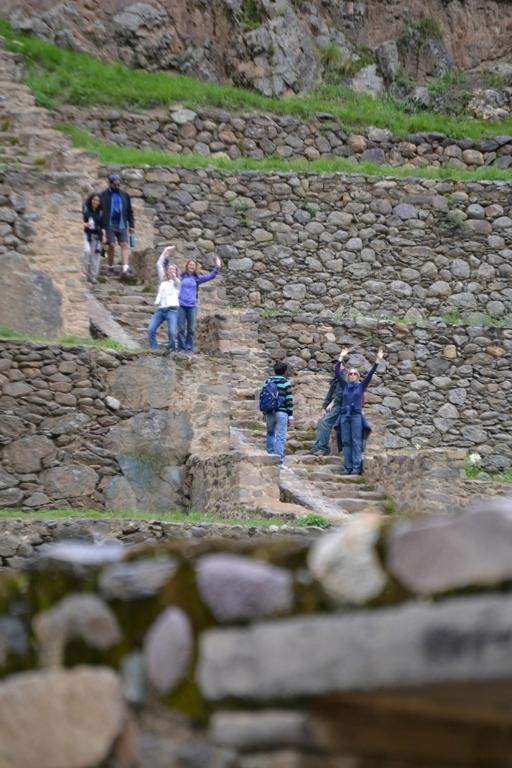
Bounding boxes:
348,373,359,376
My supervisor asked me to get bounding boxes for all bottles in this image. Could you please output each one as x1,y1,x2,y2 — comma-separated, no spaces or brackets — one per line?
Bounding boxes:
129,232,135,247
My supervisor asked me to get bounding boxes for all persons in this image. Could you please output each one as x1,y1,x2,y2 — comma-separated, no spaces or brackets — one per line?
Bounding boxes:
82,192,109,286
260,361,295,471
100,173,136,281
334,345,385,475
302,358,349,456
163,250,222,352
147,244,182,354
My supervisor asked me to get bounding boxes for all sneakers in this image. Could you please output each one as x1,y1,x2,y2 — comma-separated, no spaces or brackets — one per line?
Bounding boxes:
341,468,363,475
305,450,328,455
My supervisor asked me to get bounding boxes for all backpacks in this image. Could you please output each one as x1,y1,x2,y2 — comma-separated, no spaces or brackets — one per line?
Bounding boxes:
260,382,281,412
